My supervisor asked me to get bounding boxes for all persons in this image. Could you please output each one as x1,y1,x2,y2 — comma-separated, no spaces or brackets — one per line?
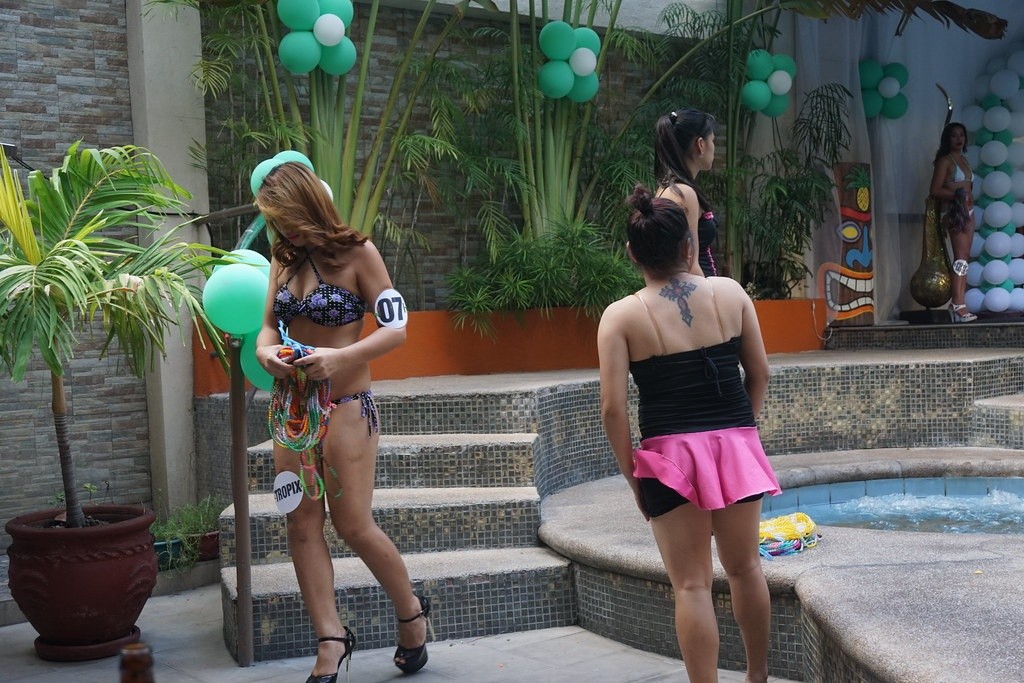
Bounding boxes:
930,124,974,323
596,185,782,683
655,108,717,277
252,162,435,683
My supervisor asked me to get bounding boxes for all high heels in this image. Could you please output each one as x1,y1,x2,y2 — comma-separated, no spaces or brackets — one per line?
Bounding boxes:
305,626,355,683
394,593,437,673
947,302,978,323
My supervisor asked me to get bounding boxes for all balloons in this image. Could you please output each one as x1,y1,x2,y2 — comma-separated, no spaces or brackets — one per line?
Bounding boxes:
569,47,597,76
883,63,908,88
862,89,883,117
761,92,788,117
575,27,601,57
539,20,576,60
538,60,574,99
859,59,883,89
203,150,334,392
319,0,353,28
742,80,771,111
880,91,908,118
278,30,321,74
314,13,345,45
774,54,796,80
768,70,792,95
277,0,320,30
960,50,1024,314
318,35,356,74
567,71,599,102
878,77,899,98
746,48,774,80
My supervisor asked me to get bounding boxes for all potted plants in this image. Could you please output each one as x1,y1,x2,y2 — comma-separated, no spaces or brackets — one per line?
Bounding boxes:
152,510,191,572
184,493,229,561
0,135,270,661
